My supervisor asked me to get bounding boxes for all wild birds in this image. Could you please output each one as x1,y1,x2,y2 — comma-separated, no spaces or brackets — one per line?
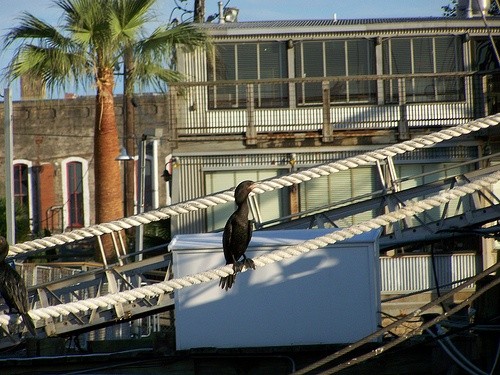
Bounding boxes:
0,235,37,336
219,180,263,292
